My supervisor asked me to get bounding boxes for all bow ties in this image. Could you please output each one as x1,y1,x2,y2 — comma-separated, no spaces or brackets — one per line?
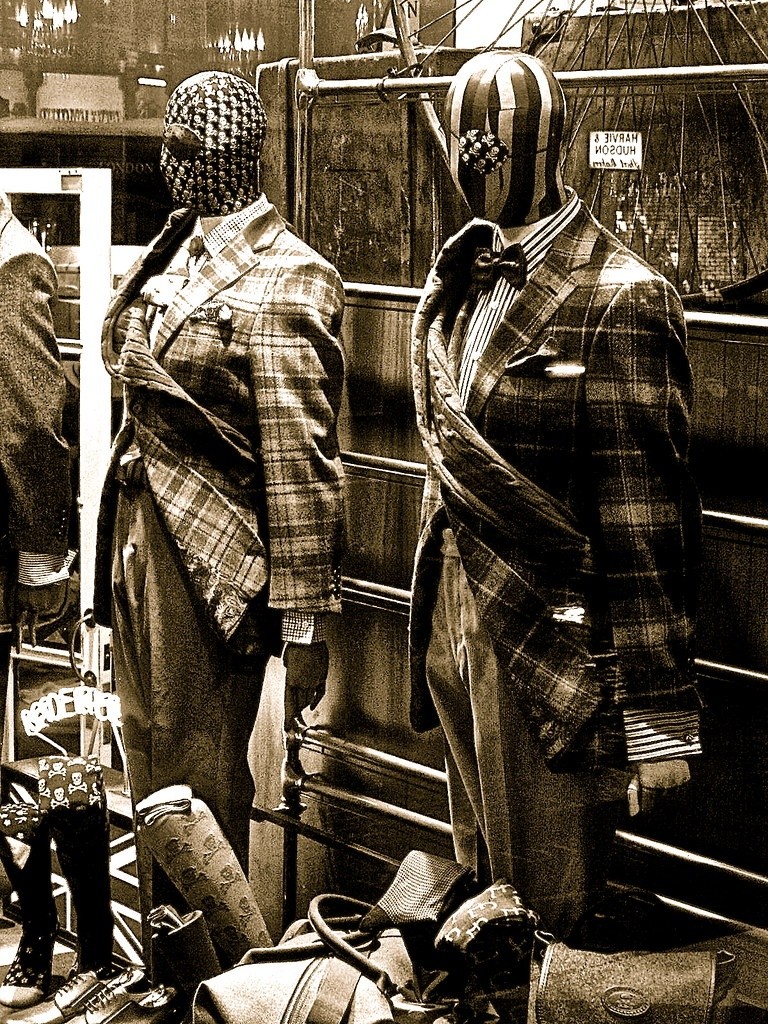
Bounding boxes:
470,242,528,292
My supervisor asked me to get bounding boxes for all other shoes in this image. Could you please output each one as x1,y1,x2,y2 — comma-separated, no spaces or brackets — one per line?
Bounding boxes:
0,911,60,1006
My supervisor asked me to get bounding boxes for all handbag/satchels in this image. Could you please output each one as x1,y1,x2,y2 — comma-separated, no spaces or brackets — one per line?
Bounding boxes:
193,893,450,1024
531,930,737,1024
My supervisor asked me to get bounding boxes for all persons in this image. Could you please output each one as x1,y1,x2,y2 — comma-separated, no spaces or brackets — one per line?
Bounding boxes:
0,181,78,1006
403,48,694,942
91,68,340,996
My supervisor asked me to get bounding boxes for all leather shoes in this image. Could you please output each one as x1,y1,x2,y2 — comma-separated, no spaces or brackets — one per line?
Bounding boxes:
62,967,176,1024
1,969,120,1024
